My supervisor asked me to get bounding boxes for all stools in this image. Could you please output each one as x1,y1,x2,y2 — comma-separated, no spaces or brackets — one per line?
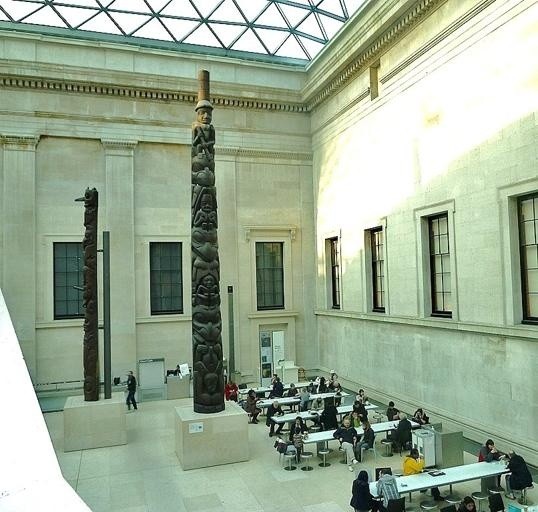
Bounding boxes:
420,501,439,512
518,485,534,505
445,495,462,510
472,492,489,512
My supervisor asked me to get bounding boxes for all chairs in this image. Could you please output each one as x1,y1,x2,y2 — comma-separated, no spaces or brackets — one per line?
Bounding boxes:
440,505,456,512
488,493,504,512
379,496,405,512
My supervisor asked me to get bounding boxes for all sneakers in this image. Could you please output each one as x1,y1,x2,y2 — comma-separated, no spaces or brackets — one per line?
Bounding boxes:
348,466,353,472
252,418,259,424
269,431,283,437
434,496,444,501
352,459,358,464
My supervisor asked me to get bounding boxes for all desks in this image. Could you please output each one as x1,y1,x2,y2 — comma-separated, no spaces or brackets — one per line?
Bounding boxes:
238,381,513,499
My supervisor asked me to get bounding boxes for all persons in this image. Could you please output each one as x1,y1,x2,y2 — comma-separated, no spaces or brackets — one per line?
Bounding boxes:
478,439,533,500
174,365,181,376
402,448,445,501
126,371,137,411
456,496,477,512
386,402,428,454
350,468,400,512
225,374,375,472
